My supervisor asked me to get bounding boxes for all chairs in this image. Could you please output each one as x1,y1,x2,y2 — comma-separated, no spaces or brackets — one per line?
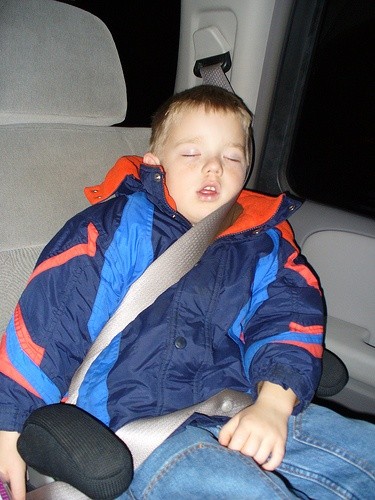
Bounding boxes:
0,0,349,500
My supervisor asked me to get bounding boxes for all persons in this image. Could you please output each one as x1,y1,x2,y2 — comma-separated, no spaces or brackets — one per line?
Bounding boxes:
1,83,375,500
